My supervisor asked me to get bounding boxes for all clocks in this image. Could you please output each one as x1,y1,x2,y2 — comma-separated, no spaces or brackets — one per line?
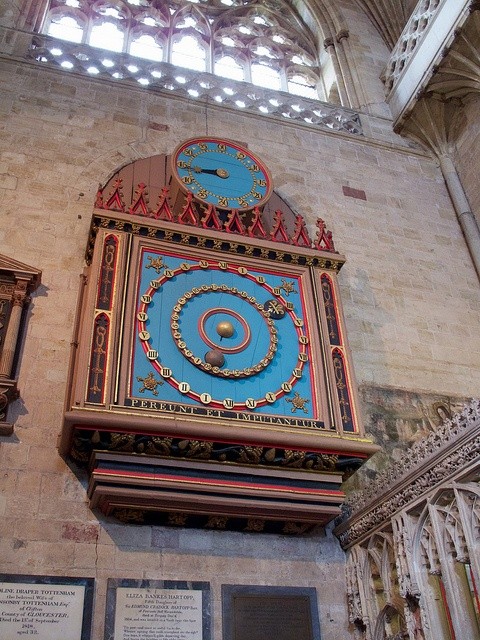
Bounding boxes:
170,135,274,213
